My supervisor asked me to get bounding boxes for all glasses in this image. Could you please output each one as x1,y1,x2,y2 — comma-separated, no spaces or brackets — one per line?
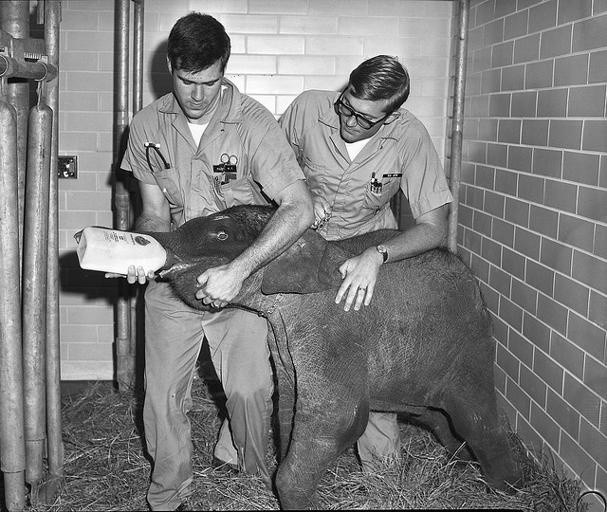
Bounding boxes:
333,82,393,131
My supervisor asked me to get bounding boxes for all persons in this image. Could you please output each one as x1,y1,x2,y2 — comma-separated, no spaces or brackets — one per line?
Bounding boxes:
103,13,315,512
211,54,456,479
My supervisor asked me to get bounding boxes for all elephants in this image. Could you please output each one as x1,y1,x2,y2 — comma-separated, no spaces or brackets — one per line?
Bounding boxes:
71,202,527,512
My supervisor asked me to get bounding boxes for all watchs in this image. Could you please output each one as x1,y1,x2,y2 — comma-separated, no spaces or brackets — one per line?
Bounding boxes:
376,243,389,265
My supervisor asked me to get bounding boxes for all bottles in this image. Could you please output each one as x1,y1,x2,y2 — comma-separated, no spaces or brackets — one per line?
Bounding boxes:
70,229,178,275
